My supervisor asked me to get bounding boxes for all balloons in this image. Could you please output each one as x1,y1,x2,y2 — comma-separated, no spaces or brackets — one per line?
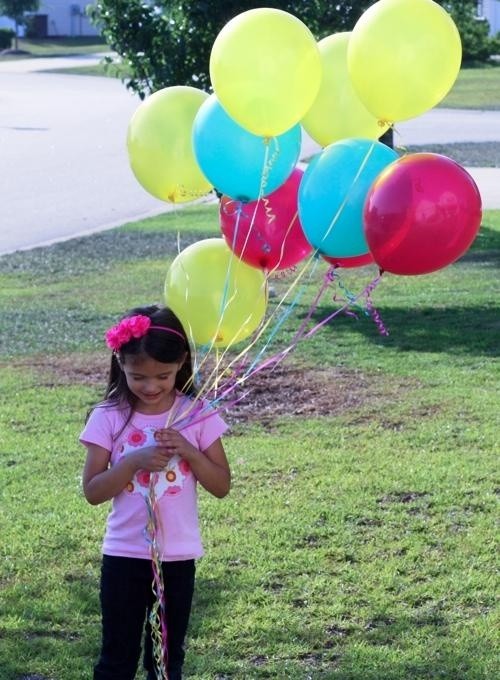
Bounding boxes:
165,238,268,349
126,86,215,201
192,8,397,275
362,151,481,277
346,2,462,122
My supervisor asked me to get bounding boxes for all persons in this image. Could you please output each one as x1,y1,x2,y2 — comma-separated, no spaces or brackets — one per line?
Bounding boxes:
79,306,230,680
